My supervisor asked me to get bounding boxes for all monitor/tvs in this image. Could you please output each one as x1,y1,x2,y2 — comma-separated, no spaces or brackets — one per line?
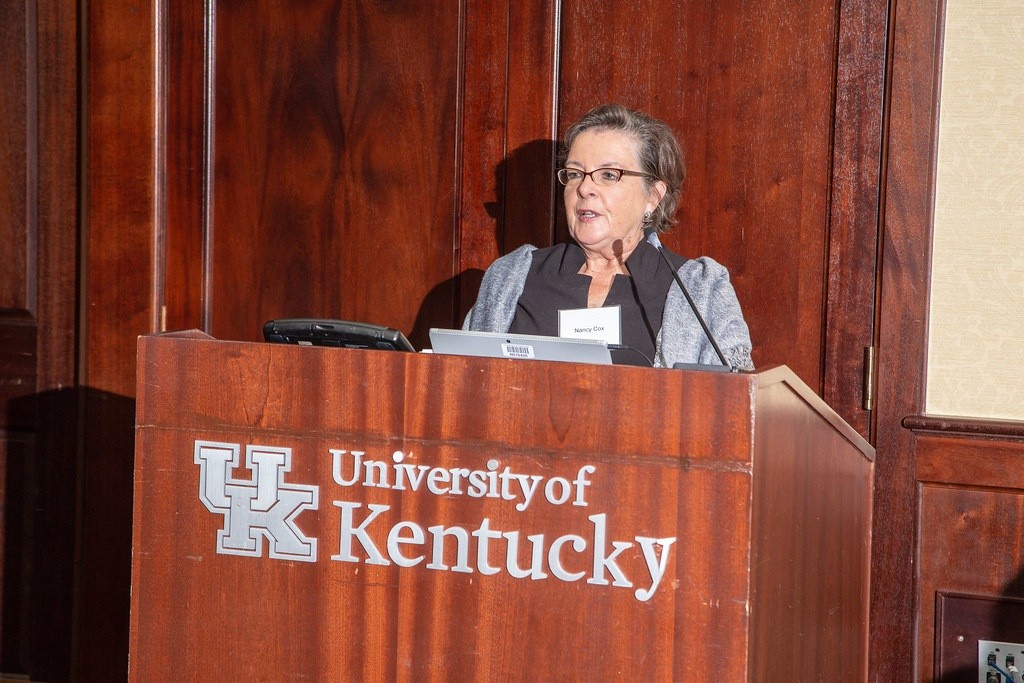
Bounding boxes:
263,317,416,353
429,327,612,365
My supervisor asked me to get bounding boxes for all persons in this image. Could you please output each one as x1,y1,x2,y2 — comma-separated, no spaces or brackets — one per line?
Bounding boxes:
462,102,756,370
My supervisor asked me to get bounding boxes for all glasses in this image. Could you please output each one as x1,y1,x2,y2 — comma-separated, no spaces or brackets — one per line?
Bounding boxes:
557,167,654,187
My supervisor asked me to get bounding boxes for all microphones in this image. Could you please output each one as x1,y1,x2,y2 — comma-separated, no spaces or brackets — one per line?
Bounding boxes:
644,226,732,371
